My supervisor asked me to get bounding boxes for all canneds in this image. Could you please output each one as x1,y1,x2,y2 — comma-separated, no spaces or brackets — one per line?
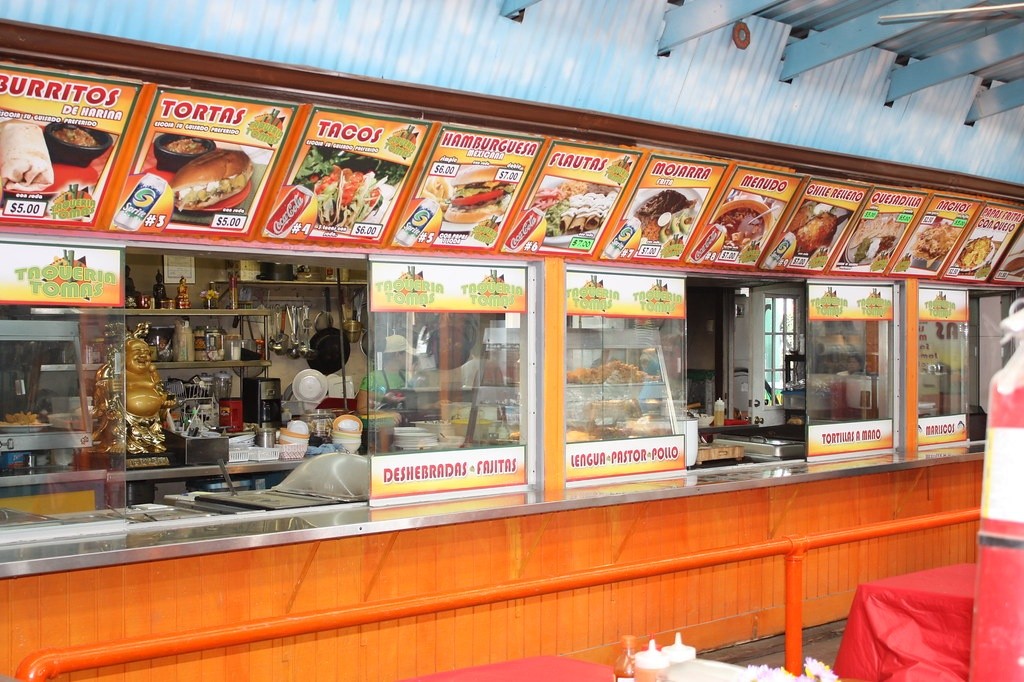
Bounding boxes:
265,185,314,237
765,232,796,269
690,223,723,264
393,197,439,248
605,217,641,259
504,207,544,252
113,172,167,232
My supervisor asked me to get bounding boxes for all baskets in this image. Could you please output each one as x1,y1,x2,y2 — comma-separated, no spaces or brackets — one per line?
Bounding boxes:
229,448,250,463
248,447,279,462
274,443,307,461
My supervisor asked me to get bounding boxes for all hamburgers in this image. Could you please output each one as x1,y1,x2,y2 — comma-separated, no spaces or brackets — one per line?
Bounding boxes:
443,164,516,224
169,147,253,211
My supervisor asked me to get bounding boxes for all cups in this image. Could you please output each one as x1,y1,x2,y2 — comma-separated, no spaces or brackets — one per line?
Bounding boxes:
23,454,37,469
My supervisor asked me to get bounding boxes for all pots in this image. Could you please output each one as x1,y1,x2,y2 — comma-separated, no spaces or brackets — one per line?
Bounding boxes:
256,262,293,281
307,287,350,374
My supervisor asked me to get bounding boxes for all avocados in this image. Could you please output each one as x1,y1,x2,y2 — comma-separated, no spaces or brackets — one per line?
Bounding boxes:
659,216,688,243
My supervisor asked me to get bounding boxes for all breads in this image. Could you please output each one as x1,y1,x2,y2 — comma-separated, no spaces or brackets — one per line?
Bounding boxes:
0,411,43,426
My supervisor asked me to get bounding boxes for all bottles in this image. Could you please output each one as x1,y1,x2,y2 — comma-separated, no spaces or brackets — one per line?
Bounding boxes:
10,348,27,407
714,397,724,427
281,408,292,427
633,639,669,682
661,632,697,665
614,635,639,682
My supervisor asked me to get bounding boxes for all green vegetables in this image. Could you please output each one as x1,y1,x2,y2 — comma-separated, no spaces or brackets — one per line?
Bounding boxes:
292,145,406,192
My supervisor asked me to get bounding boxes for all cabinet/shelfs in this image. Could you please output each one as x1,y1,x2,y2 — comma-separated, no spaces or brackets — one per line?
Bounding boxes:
40,308,273,373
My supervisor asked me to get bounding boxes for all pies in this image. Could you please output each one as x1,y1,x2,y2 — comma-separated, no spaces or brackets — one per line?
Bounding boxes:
559,214,605,234
312,167,388,228
0,123,54,191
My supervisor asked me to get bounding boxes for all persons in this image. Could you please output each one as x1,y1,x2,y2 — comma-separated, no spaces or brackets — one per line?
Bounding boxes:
835,354,864,376
357,333,419,454
92,336,171,454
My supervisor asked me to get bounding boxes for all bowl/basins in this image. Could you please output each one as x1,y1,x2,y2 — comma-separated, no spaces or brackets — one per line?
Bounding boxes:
229,435,255,448
46,122,113,168
153,133,216,172
279,420,310,445
331,415,363,453
451,420,491,441
439,436,465,448
697,416,715,425
712,200,773,256
426,313,470,370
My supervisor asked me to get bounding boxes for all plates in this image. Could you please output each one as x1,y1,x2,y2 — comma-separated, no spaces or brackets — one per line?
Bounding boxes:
627,188,703,252
845,214,904,265
394,421,451,451
955,239,995,272
284,369,327,402
1000,254,1024,275
144,167,252,211
327,374,354,397
2,126,113,195
0,424,52,433
440,213,505,233
542,180,600,246
813,202,847,251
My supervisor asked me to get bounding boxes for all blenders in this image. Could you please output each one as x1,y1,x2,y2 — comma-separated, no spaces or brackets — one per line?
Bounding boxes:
197,373,213,411
215,371,232,409
